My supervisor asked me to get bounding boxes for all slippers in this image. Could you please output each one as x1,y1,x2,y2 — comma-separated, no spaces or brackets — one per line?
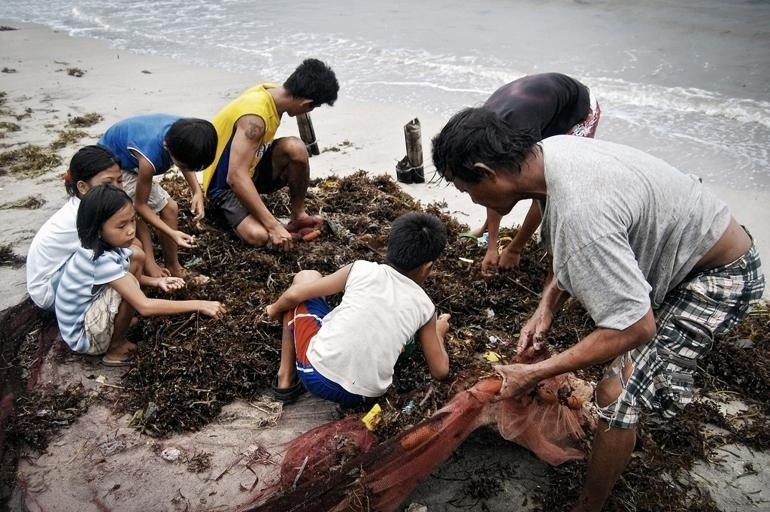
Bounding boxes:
101,356,139,367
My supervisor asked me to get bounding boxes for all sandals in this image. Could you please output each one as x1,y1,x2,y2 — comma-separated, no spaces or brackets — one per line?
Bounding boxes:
272,373,303,403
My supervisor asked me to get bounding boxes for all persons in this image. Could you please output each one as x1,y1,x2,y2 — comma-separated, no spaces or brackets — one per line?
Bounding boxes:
263,210,454,407
53,183,228,370
428,106,764,512
95,112,219,287
457,70,602,279
24,144,144,314
200,57,341,253
